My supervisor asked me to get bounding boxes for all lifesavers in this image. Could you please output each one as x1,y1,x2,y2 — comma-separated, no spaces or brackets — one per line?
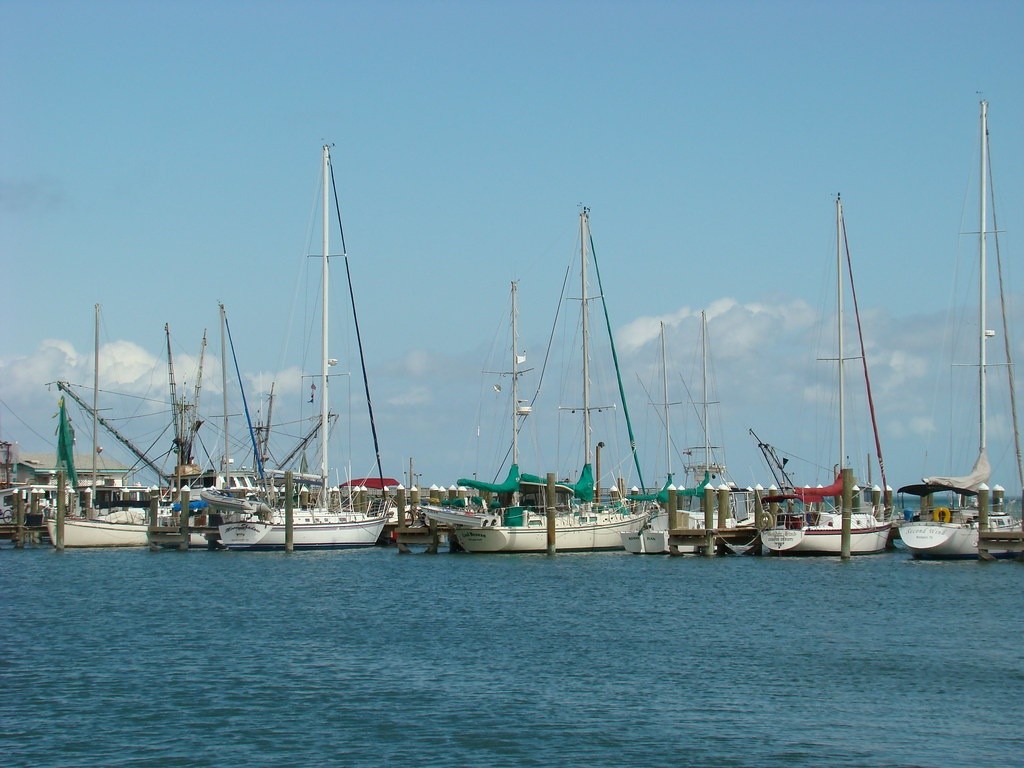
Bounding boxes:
755,509,775,529
932,505,951,522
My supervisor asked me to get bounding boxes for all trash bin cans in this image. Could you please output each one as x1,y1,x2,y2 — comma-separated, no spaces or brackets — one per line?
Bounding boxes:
904,508,913,520
24,514,44,526
208,514,224,525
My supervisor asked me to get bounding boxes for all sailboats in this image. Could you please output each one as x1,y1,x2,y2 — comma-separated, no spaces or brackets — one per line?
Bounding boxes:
1,96,1024,559
